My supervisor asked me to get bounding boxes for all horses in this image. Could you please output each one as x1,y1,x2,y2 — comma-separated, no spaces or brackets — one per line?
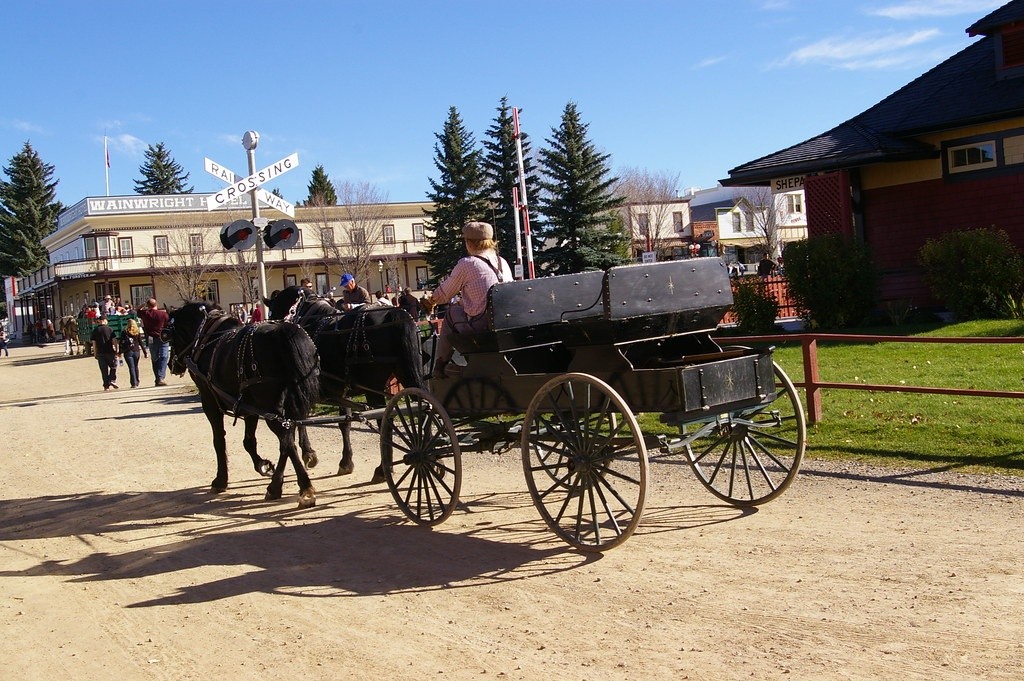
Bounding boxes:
163,302,320,510
262,286,422,483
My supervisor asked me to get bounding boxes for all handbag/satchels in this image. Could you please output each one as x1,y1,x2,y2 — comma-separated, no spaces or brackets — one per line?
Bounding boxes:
161,328,172,343
121,332,135,354
4,338,11,344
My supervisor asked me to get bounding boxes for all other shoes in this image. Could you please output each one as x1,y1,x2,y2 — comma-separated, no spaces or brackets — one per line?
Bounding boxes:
109,382,119,389
431,368,445,377
104,387,109,391
131,385,136,389
155,379,166,386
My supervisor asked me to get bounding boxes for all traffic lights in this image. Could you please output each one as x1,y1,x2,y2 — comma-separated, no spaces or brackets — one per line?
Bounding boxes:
269,220,301,249
227,220,258,250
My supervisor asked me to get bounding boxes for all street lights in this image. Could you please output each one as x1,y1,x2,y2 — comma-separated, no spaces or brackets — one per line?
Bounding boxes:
377,260,384,294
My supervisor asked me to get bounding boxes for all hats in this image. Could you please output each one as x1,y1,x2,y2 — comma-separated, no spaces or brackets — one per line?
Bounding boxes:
92,302,99,306
340,273,354,287
104,295,111,299
461,222,493,240
100,316,109,320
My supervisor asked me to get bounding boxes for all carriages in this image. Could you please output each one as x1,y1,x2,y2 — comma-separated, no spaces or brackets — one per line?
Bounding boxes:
60,314,139,357
160,256,807,555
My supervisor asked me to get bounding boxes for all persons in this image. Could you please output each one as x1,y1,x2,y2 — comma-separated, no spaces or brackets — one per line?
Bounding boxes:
30,273,434,393
0,322,9,360
422,221,517,380
737,261,746,276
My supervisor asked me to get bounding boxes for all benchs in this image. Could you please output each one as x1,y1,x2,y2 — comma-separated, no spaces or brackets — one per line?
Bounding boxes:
447,255,735,354
